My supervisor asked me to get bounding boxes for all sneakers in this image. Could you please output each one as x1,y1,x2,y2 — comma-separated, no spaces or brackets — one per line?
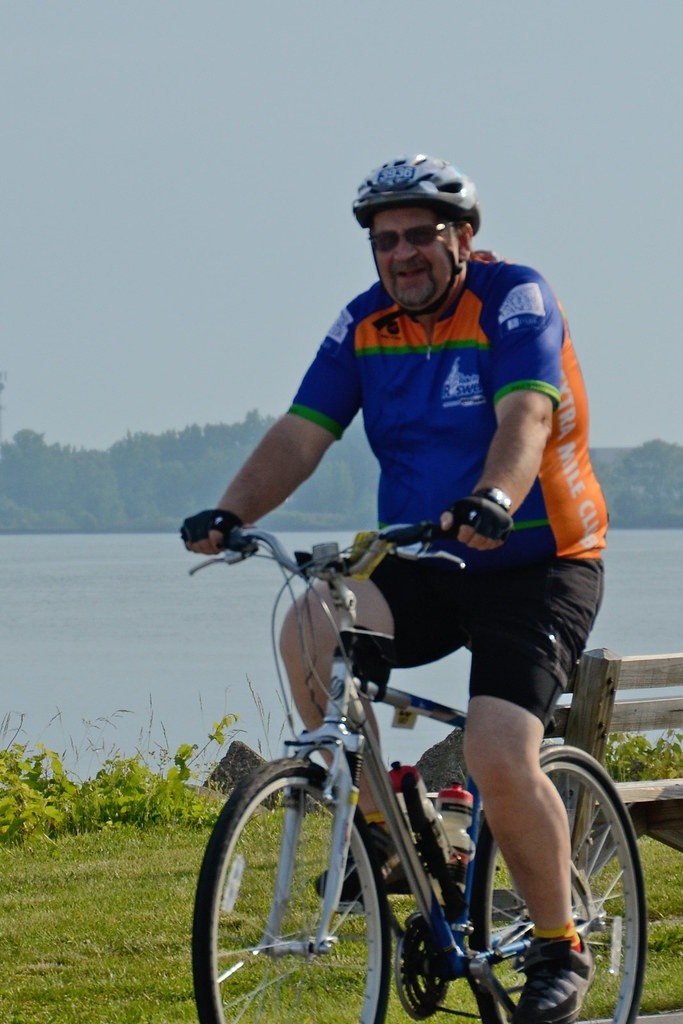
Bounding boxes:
314,860,360,903
516,932,596,1024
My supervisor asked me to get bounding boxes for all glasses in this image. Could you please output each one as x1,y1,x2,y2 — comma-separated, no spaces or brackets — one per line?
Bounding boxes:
369,222,455,251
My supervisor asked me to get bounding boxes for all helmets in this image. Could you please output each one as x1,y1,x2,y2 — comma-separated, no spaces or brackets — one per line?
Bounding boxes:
352,153,480,235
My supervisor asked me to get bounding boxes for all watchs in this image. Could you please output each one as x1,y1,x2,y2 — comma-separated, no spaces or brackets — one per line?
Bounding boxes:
476,487,511,510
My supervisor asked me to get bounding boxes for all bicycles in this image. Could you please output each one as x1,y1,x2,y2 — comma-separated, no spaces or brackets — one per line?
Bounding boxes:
187,507,649,1024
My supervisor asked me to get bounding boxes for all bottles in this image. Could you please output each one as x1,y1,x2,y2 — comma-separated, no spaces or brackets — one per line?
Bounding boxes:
436,781,473,906
387,761,450,871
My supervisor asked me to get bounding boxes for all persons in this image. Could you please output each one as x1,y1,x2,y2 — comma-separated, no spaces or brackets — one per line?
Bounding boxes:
183,154,613,1021
